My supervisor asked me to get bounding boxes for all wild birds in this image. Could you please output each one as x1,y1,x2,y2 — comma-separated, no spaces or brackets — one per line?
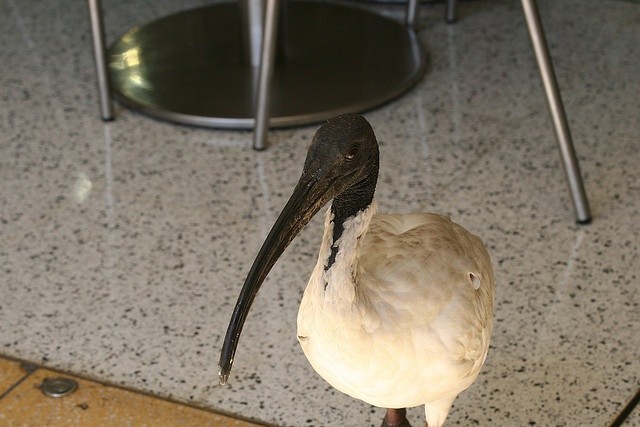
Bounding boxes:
217,110,497,427
